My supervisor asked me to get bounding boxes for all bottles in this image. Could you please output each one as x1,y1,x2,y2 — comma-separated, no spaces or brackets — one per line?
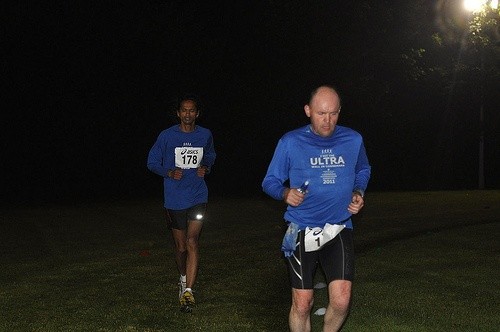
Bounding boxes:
289,179,310,208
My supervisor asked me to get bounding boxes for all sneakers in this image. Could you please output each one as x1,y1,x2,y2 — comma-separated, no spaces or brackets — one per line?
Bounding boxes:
178,275,187,299
179,289,195,311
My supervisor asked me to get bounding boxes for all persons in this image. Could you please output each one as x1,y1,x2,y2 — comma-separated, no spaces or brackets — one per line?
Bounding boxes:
147,99,217,313
262,85,372,332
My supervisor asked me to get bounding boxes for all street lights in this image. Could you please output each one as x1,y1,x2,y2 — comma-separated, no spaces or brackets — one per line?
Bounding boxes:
461,1,500,190
